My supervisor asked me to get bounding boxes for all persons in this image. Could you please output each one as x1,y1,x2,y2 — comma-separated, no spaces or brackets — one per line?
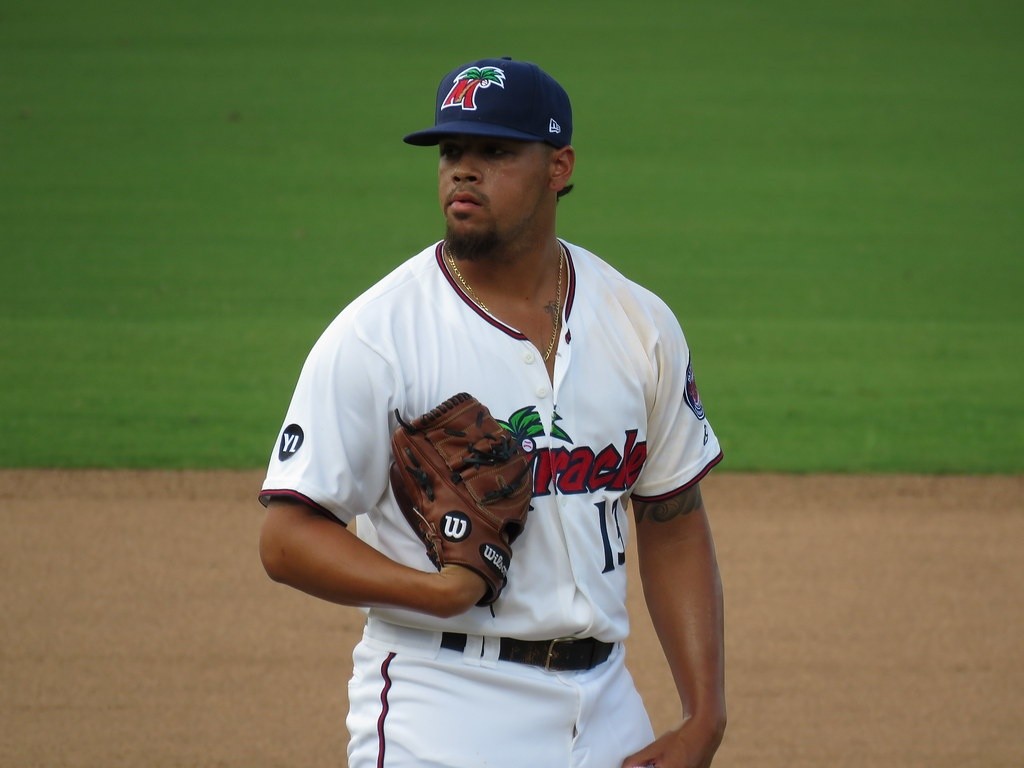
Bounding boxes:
258,55,728,768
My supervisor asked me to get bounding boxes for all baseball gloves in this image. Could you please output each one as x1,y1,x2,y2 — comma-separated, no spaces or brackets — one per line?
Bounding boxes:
388,391,535,609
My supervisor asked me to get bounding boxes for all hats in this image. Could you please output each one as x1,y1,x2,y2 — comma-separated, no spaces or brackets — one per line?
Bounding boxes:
403,55,573,150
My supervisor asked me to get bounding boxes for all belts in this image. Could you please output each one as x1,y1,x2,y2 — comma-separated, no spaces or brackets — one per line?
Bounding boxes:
439,630,616,671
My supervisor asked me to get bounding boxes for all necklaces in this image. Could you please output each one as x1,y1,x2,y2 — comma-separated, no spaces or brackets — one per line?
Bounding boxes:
444,239,563,362
